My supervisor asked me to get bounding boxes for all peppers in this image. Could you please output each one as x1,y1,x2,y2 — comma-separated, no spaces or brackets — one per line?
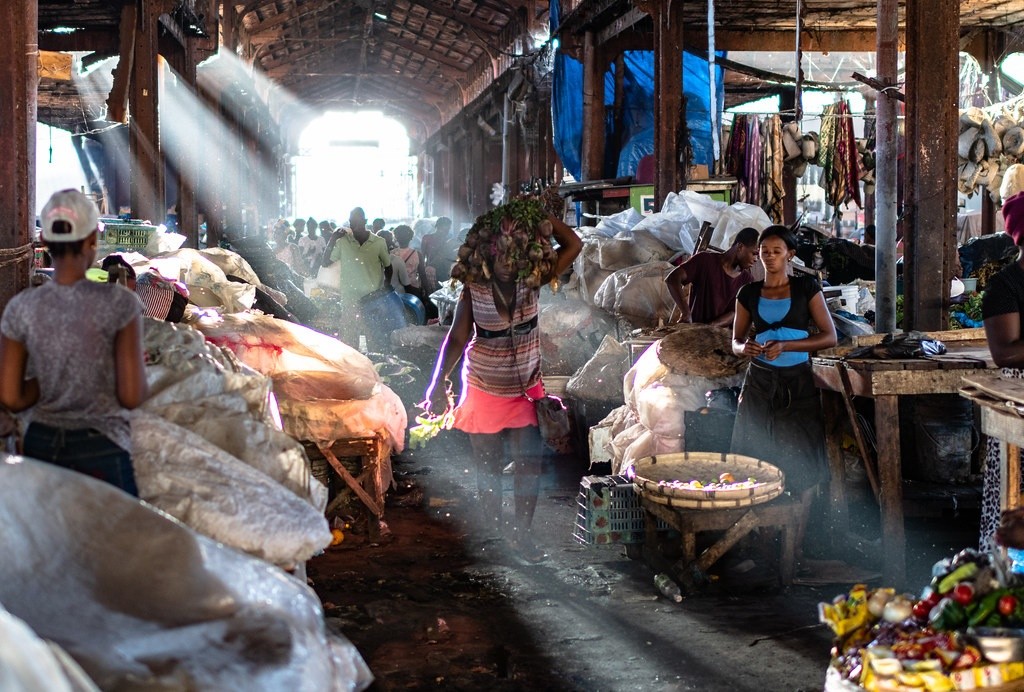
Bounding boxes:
911,561,1024,632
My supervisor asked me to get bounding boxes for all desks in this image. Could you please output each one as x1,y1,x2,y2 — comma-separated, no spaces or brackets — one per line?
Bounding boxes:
641,491,808,581
301,436,380,544
817,347,1024,594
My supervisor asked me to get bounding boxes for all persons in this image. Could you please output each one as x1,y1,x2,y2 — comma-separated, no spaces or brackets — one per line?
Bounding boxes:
981,190,1024,369
731,226,839,581
1,189,150,500
100,254,137,292
269,216,470,328
663,227,761,330
424,205,585,563
322,207,393,353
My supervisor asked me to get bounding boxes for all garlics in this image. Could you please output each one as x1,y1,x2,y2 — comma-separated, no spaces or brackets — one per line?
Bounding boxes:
867,587,912,624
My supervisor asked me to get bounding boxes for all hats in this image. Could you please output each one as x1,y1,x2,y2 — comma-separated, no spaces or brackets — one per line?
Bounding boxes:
41,188,97,242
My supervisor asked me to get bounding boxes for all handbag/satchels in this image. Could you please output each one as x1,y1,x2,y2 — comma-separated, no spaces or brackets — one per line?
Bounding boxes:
537,397,570,439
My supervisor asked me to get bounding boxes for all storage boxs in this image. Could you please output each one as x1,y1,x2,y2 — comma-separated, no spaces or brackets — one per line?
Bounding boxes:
571,475,641,548
101,218,155,250
